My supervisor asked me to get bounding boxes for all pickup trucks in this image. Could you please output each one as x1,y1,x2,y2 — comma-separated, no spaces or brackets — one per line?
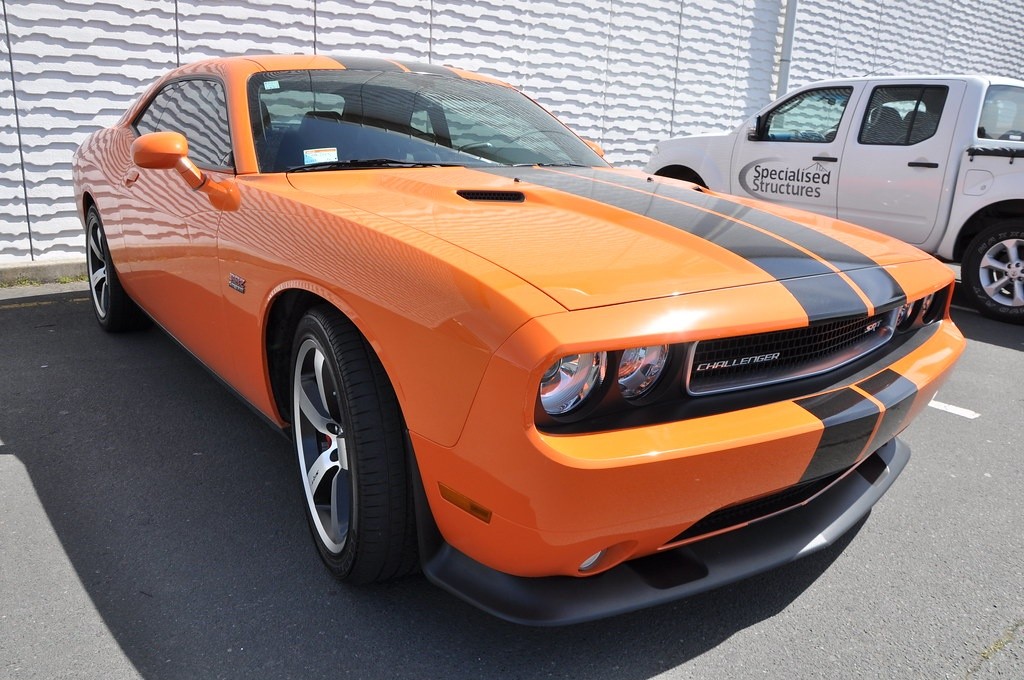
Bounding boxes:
642,74,1024,325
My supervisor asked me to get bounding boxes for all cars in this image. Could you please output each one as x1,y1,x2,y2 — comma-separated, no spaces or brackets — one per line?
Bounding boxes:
71,54,965,627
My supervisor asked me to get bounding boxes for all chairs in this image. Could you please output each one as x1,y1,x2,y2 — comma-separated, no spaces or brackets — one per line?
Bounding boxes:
254,100,440,174
861,106,930,145
